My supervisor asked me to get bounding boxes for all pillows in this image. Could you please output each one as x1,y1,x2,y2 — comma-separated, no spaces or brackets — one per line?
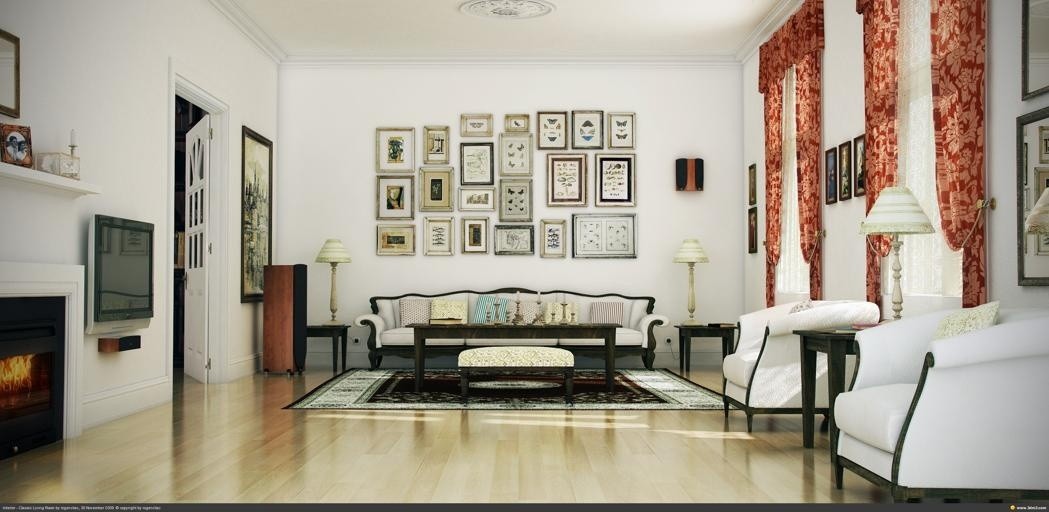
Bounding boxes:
588,301,622,327
933,301,1000,339
430,299,463,320
790,298,814,316
398,298,429,328
474,294,504,324
545,301,577,325
507,299,541,326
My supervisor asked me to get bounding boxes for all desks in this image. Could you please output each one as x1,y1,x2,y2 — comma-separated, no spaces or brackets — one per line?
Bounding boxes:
792,326,858,492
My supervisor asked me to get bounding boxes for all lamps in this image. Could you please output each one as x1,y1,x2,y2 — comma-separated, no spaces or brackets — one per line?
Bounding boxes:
862,185,935,318
316,238,351,326
674,237,711,326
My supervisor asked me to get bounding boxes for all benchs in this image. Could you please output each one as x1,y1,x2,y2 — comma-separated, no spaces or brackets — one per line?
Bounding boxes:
456,347,574,403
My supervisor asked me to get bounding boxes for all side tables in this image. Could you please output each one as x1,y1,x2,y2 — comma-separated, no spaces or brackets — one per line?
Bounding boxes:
675,326,738,376
305,326,350,376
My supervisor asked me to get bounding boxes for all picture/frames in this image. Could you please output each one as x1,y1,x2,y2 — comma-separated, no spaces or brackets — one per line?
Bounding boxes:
493,224,535,256
375,126,416,174
747,207,758,254
461,217,489,256
1019,0,1048,101
459,142,494,186
537,111,568,151
423,124,450,164
608,110,637,150
1015,108,1048,285
498,179,533,222
0,29,20,119
422,216,455,258
375,224,416,256
1,122,32,165
571,213,637,260
546,152,588,207
376,176,413,222
840,140,852,200
505,113,530,133
460,112,494,138
570,109,605,151
853,134,868,198
594,153,637,207
417,166,454,213
748,164,757,204
458,186,494,212
241,126,272,303
498,133,533,176
539,219,567,259
823,146,838,203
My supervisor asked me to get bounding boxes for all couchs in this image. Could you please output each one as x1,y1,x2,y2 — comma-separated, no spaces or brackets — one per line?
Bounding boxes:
720,301,881,434
356,288,669,373
830,305,1048,506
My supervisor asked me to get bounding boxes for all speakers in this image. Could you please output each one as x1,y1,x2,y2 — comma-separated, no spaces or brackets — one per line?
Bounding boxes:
263,264,308,377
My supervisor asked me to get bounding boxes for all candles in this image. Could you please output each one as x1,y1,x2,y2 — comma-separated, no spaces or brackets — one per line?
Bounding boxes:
70,128,77,145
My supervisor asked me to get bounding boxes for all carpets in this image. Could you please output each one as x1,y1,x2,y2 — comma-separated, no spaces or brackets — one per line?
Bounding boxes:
281,367,741,412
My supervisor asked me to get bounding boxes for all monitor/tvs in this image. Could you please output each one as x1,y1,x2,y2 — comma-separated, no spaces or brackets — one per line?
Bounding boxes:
85,214,154,333
